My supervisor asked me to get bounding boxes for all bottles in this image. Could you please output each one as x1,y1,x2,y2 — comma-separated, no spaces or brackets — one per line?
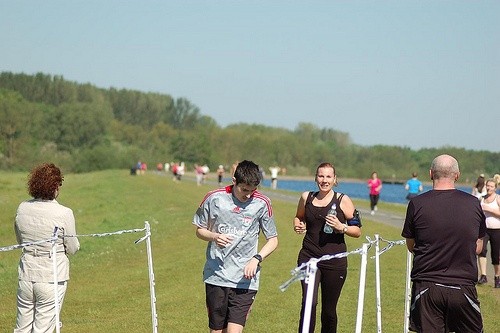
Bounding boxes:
324,204,337,233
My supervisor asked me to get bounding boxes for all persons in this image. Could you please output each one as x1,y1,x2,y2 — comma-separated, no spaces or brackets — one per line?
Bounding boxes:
13,164,80,333
191,160,278,333
368,170,382,216
294,163,363,333
126,151,283,191
398,165,500,288
402,154,488,333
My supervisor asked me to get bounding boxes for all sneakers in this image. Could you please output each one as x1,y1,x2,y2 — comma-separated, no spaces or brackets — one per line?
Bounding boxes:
494,275,500,288
478,274,487,284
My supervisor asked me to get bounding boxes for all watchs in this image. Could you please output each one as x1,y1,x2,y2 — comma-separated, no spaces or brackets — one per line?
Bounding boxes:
340,224,349,234
253,255,263,263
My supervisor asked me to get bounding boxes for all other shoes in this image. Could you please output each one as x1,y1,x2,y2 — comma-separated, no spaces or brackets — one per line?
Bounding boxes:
374,206,379,212
371,210,374,215
